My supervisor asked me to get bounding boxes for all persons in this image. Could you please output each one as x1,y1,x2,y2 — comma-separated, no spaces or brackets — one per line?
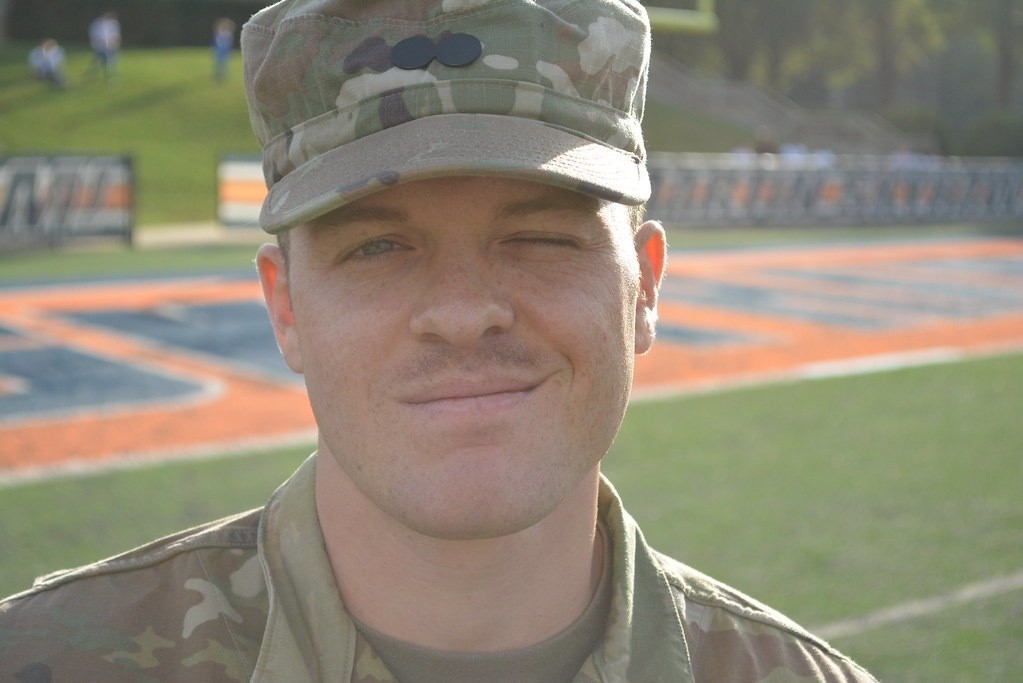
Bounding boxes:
82,11,121,92
29,37,65,93
0,1,882,683
212,16,234,82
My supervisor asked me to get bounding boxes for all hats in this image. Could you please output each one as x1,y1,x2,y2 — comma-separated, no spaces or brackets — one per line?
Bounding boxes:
240,0,652,235
